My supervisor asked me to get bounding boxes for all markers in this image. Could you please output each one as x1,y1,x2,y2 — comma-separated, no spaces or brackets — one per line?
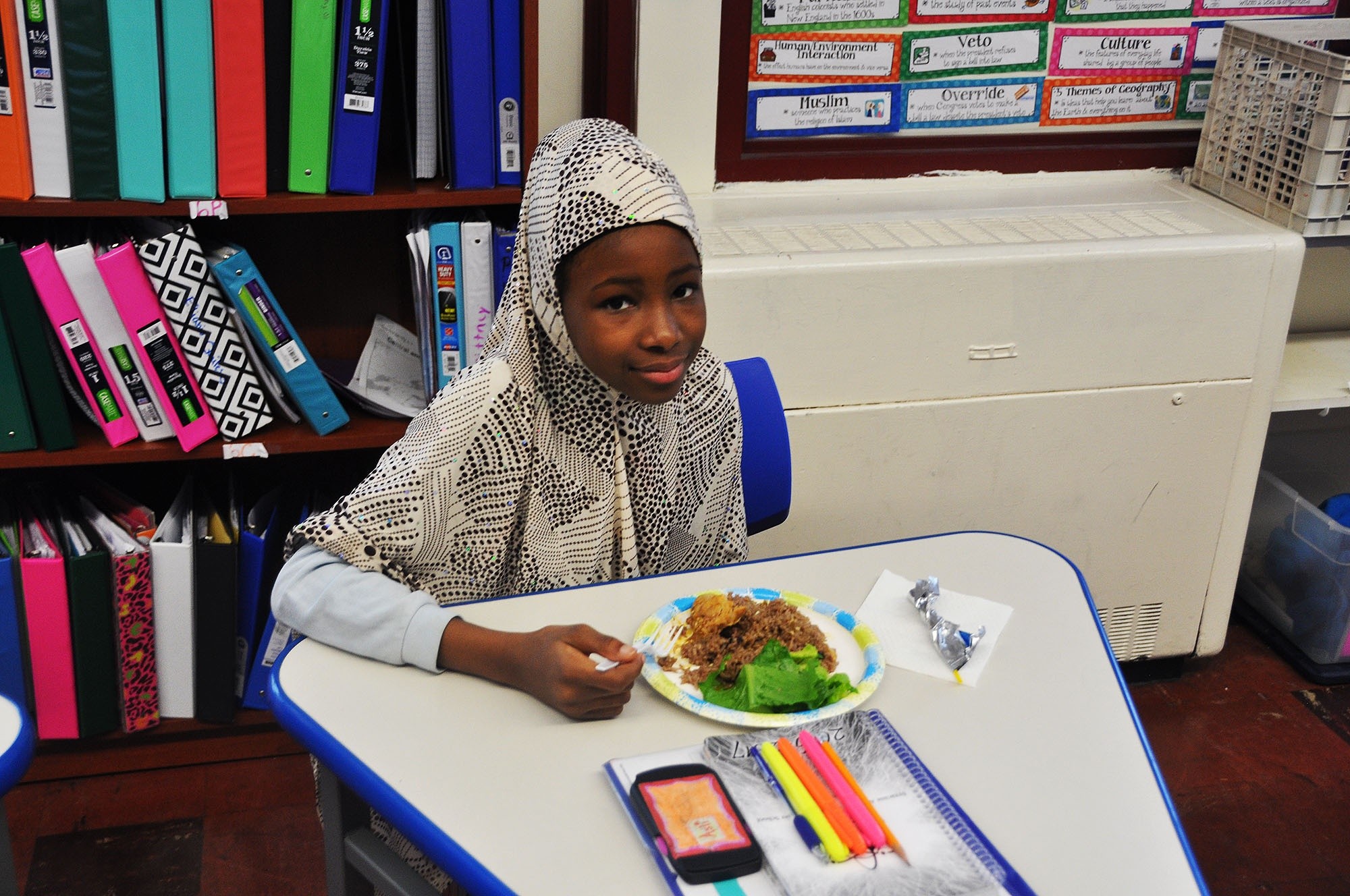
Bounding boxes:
796,729,886,851
759,741,847,864
777,738,865,857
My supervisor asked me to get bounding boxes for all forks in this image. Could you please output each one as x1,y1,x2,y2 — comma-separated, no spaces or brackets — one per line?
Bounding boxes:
595,624,684,672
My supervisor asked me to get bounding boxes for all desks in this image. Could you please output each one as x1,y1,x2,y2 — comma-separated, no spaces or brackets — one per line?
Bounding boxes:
270,527,1210,896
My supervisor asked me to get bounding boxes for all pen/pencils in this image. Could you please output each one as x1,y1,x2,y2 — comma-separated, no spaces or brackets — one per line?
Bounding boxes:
817,733,913,869
746,745,829,867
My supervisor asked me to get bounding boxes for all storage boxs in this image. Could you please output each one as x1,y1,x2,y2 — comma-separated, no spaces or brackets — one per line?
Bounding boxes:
1191,16,1350,241
1242,417,1349,663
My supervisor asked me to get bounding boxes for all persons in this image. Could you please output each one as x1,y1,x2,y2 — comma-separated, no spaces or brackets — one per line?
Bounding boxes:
267,116,747,895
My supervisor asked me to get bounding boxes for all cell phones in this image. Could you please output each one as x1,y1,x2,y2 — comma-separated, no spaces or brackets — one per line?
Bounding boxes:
631,763,763,885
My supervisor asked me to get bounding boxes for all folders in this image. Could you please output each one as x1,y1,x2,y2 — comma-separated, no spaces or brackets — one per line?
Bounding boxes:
204,244,347,433
16,239,138,449
418,215,514,404
51,223,177,446
0,504,343,751
131,219,271,443
0,0,530,199
91,232,217,454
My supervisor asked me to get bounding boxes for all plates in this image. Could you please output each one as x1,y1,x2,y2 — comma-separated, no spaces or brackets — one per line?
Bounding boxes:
634,587,886,729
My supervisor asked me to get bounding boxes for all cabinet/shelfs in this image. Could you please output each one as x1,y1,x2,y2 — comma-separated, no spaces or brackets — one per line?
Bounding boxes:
0,194,518,469
